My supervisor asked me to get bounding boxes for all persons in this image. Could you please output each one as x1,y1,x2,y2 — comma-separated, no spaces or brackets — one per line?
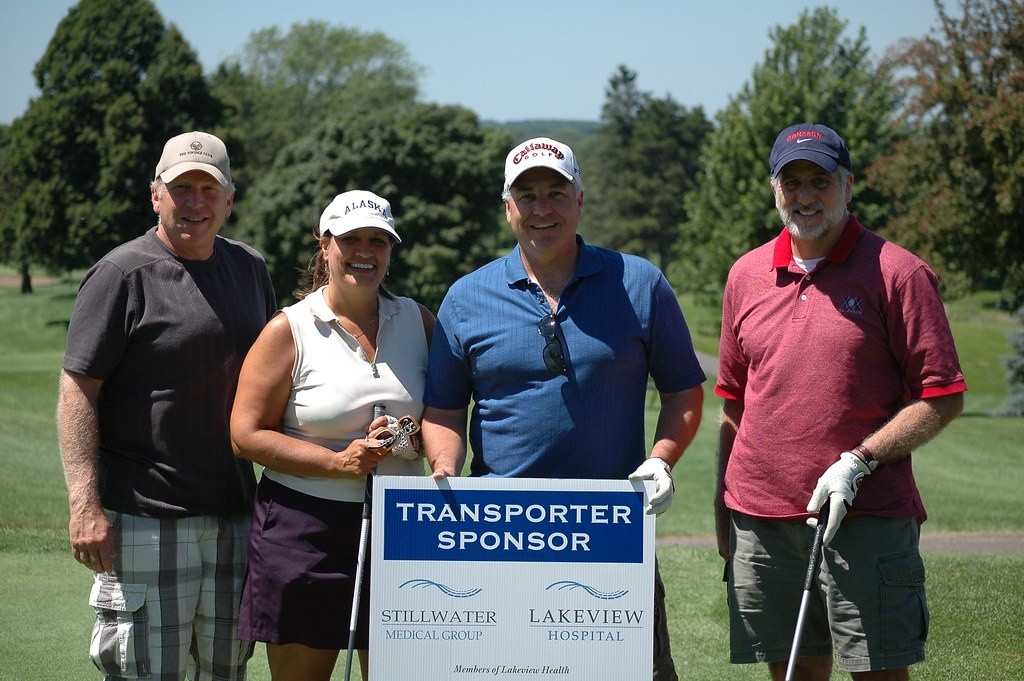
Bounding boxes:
716,122,968,680
55,131,280,680
227,188,437,681
420,139,708,680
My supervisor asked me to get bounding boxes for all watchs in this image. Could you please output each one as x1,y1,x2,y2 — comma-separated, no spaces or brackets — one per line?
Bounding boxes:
856,444,879,473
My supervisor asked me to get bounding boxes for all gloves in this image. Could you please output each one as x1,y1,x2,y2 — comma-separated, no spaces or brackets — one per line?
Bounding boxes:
628,457,674,518
805,450,872,548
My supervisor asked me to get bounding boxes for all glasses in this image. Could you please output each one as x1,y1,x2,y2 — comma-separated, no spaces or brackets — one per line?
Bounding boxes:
538,313,568,374
367,414,420,452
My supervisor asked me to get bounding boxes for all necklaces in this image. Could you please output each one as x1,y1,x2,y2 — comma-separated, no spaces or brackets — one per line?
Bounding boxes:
350,311,381,338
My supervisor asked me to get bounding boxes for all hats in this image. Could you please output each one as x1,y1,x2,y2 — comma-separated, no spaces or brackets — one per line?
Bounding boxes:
768,121,852,180
319,190,402,246
504,136,581,190
155,131,233,187
